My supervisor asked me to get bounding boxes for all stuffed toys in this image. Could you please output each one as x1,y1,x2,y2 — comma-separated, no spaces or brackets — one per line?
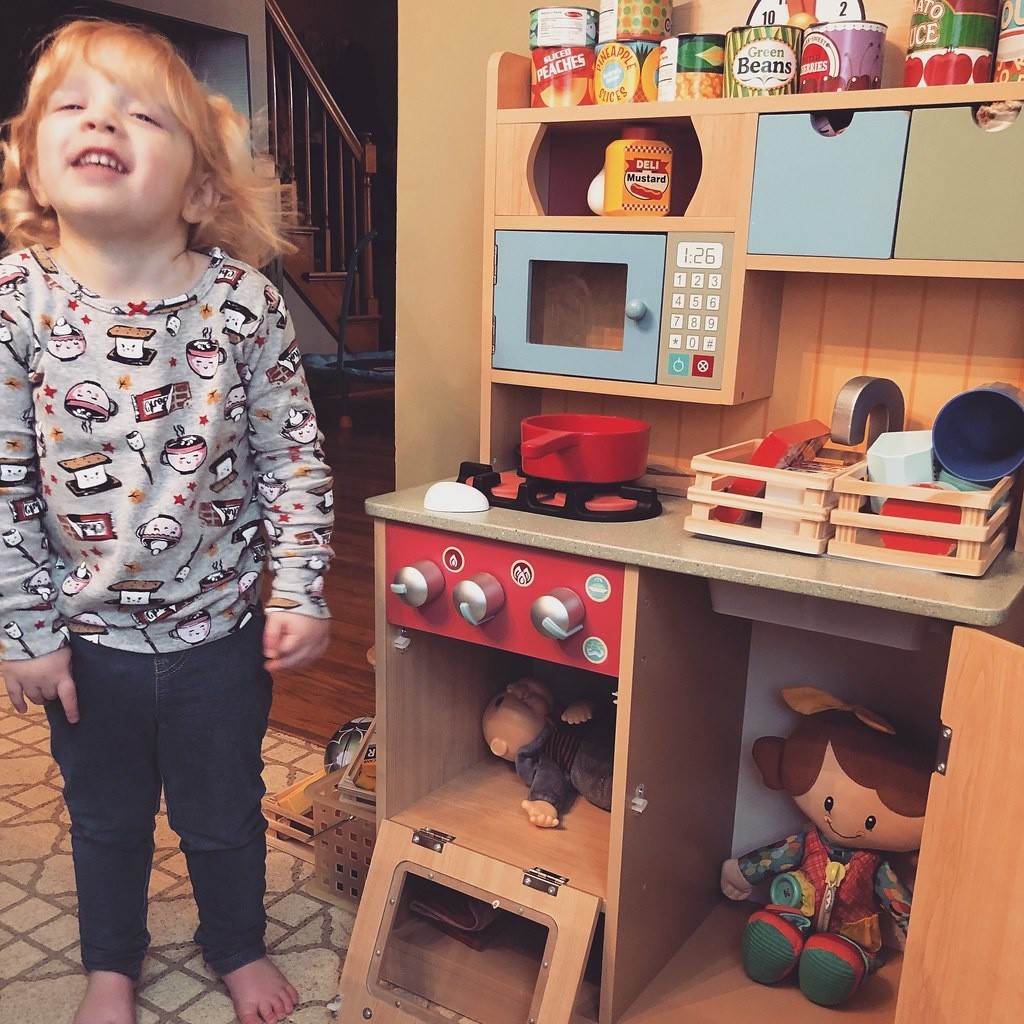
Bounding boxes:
718,686,935,1001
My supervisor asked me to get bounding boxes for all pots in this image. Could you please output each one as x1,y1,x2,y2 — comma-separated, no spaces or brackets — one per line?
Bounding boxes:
516,411,652,484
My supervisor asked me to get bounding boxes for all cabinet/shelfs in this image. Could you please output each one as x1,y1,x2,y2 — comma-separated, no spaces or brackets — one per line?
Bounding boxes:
328,51,1024,1024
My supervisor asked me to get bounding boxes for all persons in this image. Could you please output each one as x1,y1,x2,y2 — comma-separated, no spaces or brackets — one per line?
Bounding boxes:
482,669,613,831
0,14,334,1024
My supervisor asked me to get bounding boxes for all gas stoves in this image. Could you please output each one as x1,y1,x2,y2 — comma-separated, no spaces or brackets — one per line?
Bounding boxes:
383,466,665,678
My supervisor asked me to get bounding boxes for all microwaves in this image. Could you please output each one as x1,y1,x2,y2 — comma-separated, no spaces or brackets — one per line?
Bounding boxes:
489,230,781,404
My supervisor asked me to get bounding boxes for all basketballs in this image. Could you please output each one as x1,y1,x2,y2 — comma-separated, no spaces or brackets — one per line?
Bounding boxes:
324,717,374,775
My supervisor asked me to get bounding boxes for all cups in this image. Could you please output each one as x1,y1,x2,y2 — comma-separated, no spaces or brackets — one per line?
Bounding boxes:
929,379,1024,484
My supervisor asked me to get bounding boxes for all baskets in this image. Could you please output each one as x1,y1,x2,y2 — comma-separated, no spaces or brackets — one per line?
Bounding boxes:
299,765,380,914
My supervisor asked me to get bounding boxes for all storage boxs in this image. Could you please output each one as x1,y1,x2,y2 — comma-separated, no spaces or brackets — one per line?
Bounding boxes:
683,438,867,555
825,461,1020,578
